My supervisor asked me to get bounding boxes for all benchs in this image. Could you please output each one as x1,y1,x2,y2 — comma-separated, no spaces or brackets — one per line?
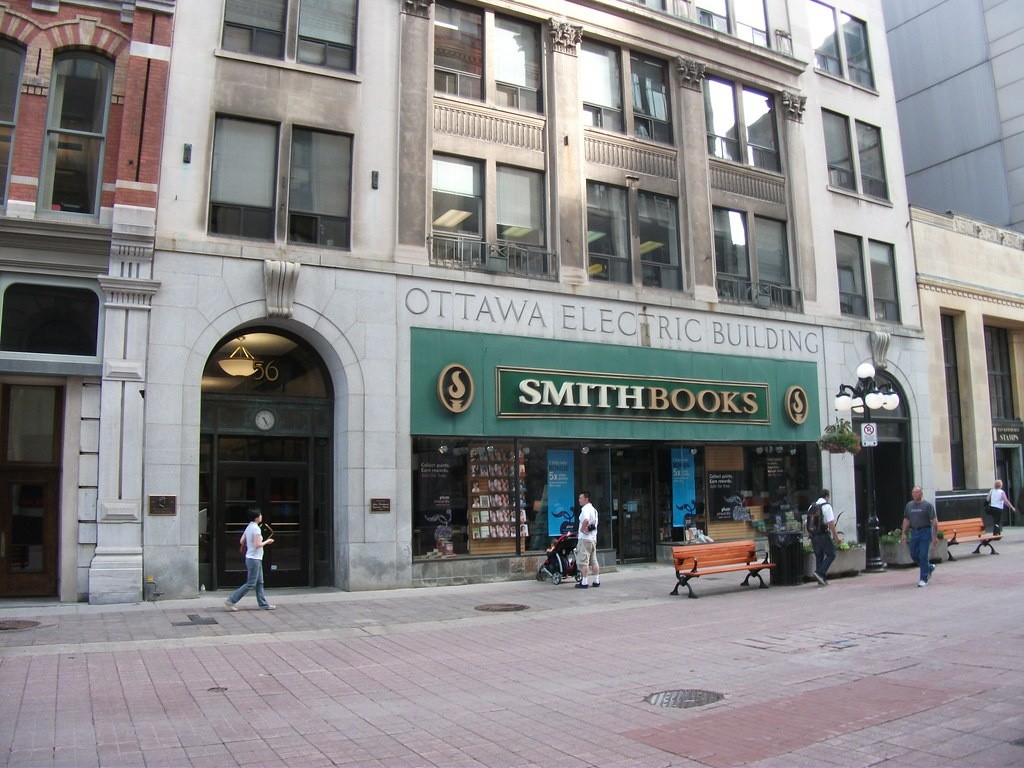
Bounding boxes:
670,539,776,599
936,517,1003,562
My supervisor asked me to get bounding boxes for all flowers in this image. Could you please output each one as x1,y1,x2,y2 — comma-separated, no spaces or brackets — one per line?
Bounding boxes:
818,417,862,454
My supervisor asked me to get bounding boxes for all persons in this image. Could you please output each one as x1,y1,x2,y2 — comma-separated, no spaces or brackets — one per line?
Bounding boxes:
986,480,1016,537
808,489,841,587
574,491,600,589
545,539,557,554
224,508,276,611
901,486,940,587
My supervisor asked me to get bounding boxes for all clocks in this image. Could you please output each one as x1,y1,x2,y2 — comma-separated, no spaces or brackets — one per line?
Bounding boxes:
254,409,275,430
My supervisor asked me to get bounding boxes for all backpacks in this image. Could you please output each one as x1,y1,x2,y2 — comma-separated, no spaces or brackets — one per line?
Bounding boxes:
807,502,831,535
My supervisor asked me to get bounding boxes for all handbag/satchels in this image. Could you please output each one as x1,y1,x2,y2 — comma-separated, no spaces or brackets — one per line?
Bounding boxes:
985,506,992,515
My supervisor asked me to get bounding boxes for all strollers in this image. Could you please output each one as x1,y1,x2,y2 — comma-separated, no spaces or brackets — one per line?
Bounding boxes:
535,530,583,585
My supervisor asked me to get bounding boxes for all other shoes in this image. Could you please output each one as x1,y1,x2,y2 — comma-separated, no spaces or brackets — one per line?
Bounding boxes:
593,582,600,587
259,605,276,610
928,564,935,578
814,571,830,585
918,580,929,587
575,583,588,588
224,600,238,612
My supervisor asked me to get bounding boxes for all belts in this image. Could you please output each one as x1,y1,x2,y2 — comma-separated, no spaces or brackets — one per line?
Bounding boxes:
912,525,930,530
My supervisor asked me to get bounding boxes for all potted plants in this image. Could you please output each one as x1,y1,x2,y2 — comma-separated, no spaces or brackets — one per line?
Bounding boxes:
803,540,867,579
879,526,948,567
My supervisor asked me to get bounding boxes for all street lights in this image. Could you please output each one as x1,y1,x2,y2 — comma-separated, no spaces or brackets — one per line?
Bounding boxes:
833,362,900,573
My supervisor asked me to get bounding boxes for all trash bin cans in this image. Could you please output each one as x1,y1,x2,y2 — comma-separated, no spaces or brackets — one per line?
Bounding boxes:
767,530,803,586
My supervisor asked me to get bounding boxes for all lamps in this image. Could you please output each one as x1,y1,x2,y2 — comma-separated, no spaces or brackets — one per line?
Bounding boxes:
486,442,494,452
579,442,589,454
522,441,530,454
689,447,698,455
788,446,796,455
218,336,265,378
439,441,448,454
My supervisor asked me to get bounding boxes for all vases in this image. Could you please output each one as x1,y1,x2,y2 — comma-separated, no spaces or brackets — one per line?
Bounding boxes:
829,448,845,453
